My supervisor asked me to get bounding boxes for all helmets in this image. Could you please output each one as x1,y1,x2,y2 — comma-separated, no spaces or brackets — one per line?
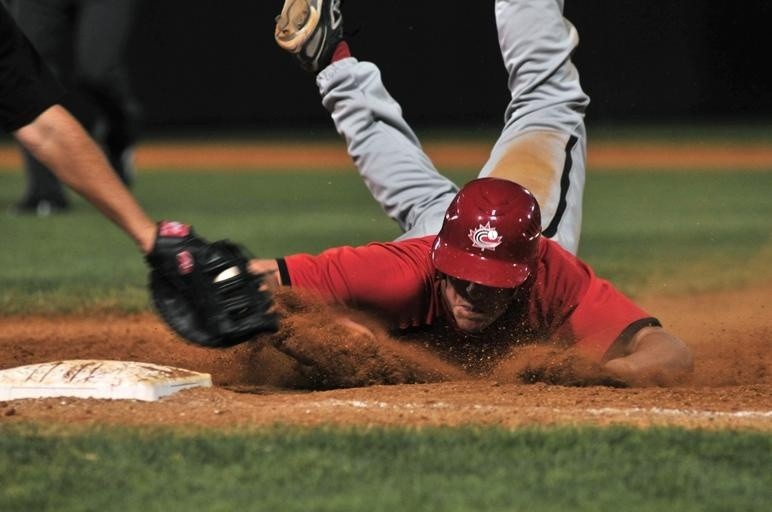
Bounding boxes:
430,178,542,289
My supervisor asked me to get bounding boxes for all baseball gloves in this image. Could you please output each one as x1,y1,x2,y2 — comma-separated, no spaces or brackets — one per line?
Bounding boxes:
146,223,275,349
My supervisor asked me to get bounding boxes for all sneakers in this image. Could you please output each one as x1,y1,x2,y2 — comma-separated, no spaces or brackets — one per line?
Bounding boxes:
274,0,344,76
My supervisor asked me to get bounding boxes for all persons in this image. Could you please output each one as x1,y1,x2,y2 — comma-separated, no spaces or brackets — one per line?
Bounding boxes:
1,0,137,218
0,6,281,351
237,2,694,396
